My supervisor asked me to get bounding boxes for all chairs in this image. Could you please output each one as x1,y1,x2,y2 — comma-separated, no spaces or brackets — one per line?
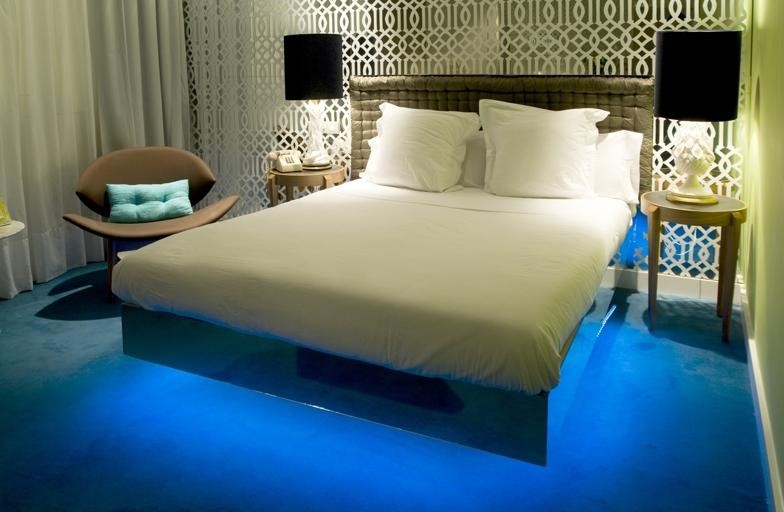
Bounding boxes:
62,145,240,302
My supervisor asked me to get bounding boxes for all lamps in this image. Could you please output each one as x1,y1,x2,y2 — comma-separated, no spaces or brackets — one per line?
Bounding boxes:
283,33,343,170
654,29,741,206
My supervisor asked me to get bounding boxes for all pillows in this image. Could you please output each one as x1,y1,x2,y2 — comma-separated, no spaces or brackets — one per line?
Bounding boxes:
359,101,479,192
478,96,610,198
366,130,486,188
595,129,643,204
105,179,194,223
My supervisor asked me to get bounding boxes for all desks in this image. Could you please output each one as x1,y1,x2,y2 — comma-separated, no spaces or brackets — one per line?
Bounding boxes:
0,219,26,239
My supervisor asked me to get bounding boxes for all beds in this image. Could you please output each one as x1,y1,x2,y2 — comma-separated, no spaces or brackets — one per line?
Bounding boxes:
111,73,655,399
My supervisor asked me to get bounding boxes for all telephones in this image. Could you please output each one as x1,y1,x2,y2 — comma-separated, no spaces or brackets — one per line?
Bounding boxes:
266,149,302,172
